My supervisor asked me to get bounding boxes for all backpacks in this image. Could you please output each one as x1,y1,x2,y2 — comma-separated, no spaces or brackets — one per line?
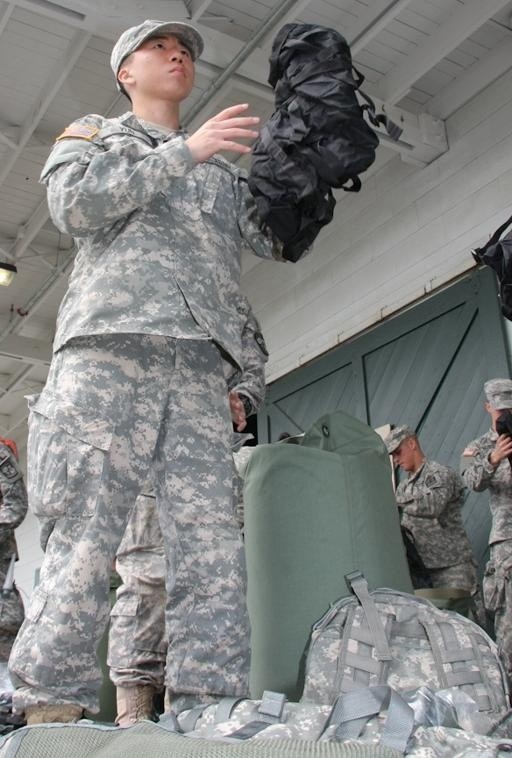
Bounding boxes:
169,571,512,758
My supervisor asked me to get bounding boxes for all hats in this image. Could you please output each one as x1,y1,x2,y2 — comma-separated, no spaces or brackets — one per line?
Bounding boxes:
386,424,416,454
110,17,204,94
483,377,512,412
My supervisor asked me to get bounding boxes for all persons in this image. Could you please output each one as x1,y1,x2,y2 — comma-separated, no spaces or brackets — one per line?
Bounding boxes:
2,15,313,733
107,294,268,731
461,376,511,675
1,434,32,657
387,424,486,642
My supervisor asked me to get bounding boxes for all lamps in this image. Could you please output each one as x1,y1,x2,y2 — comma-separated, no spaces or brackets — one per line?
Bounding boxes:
1,262,18,287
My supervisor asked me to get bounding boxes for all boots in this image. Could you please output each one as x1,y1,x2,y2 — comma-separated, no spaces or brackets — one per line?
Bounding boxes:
112,685,162,731
